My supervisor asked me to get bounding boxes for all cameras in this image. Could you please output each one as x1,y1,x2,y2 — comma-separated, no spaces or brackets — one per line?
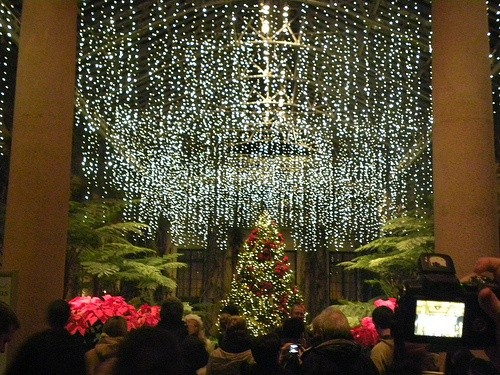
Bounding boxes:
394,253,500,348
288,344,301,354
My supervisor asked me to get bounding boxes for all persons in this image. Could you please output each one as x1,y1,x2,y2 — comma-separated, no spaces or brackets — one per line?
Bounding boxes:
0,257,500,375
